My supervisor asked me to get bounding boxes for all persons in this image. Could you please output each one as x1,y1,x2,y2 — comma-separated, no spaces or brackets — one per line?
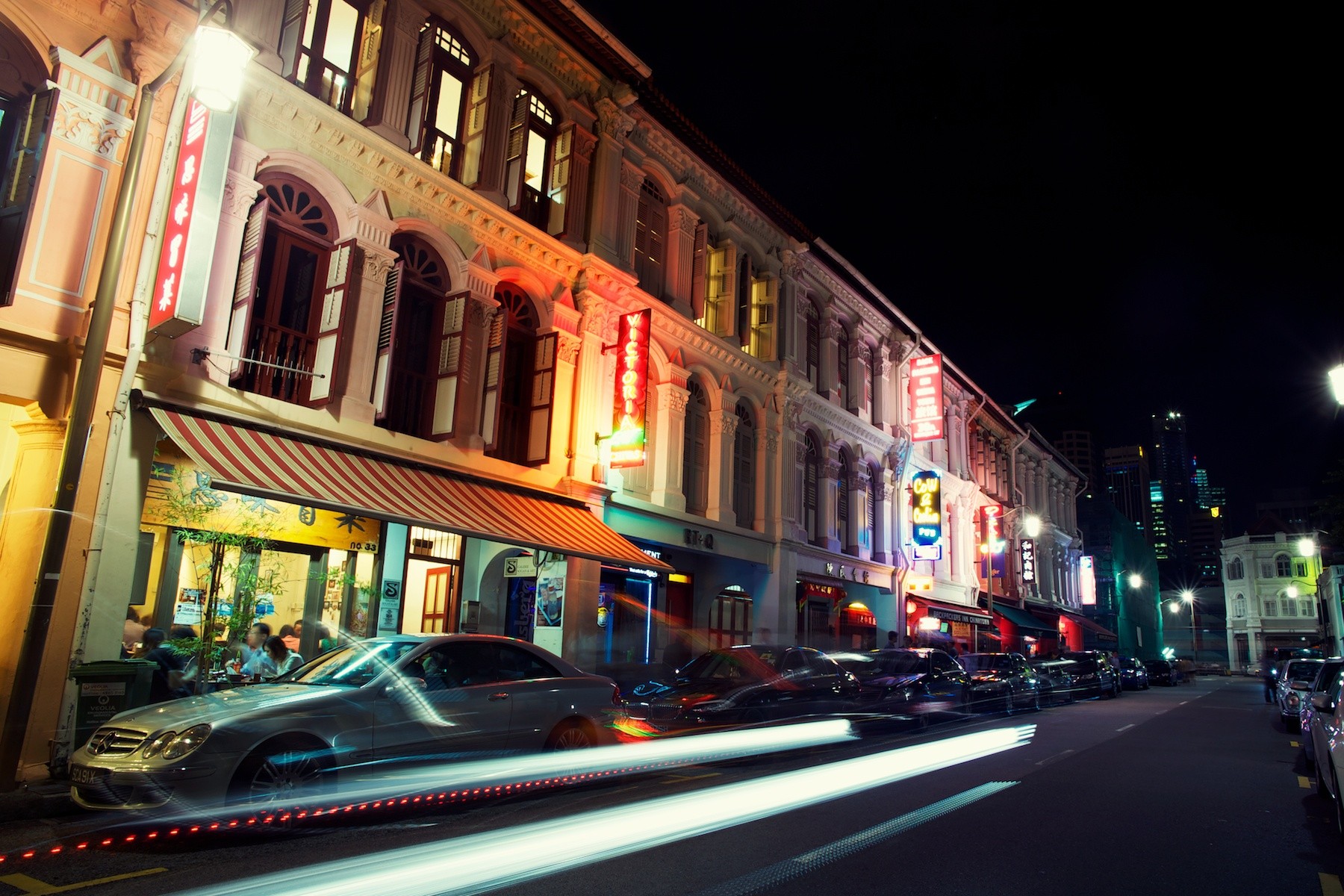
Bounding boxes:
885,630,1040,659
1109,651,1121,693
750,628,776,646
1261,650,1279,705
662,629,692,671
120,605,339,708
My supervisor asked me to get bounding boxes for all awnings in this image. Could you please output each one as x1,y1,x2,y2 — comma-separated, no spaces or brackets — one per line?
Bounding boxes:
907,591,1117,637
149,404,676,573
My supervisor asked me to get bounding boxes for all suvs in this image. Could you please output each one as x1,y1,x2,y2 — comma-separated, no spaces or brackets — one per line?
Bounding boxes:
1265,647,1323,704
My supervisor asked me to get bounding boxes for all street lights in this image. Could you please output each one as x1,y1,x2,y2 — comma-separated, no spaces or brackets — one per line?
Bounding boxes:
1116,569,1141,647
1287,579,1328,659
987,506,1039,653
1181,592,1197,668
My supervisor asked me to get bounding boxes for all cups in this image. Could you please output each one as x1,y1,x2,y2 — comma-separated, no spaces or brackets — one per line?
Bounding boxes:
541,586,548,609
253,665,263,681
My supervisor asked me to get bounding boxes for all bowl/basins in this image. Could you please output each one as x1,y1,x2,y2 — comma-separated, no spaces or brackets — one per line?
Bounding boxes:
556,590,563,600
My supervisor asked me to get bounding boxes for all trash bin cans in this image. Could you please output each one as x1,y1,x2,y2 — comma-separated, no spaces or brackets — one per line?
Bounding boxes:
70,658,162,748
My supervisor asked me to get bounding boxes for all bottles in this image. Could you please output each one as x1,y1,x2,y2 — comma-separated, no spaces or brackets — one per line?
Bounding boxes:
547,584,558,620
234,657,241,673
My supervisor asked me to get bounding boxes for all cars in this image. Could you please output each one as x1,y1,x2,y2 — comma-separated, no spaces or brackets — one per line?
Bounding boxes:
65,636,631,846
1248,663,1262,678
628,643,1176,733
1300,659,1344,833
1275,658,1325,722
